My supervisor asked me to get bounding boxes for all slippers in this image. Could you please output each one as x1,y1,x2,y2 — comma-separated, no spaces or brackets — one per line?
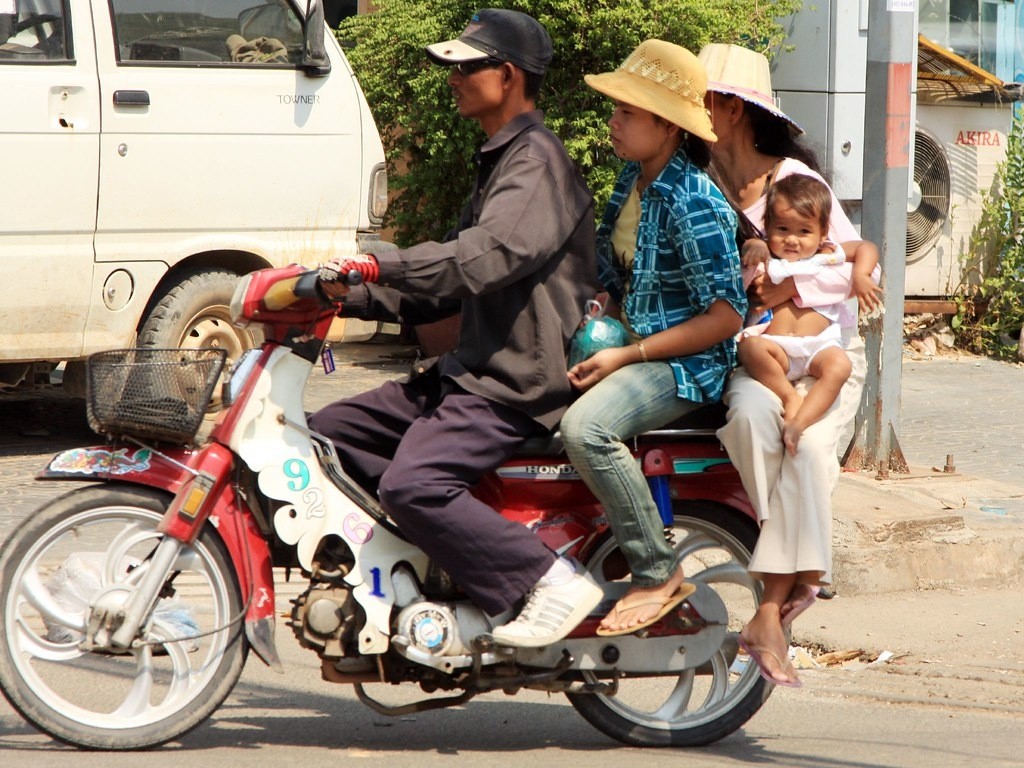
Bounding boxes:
737,636,801,687
782,587,819,625
596,583,696,637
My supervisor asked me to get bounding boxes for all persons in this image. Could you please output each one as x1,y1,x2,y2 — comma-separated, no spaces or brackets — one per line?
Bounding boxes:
560,40,746,636
325,9,606,647
735,174,884,456
694,43,880,688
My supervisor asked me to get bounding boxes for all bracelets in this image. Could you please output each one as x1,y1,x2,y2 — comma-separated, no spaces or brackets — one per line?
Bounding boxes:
634,341,647,362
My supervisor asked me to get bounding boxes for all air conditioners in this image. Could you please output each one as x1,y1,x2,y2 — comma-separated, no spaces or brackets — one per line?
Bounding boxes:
905,100,1013,296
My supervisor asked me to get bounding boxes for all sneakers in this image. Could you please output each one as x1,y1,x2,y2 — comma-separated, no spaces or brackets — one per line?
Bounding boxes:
492,556,604,648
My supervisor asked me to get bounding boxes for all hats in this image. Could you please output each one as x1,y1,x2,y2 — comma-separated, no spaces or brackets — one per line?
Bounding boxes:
583,38,719,143
424,8,553,77
697,44,807,137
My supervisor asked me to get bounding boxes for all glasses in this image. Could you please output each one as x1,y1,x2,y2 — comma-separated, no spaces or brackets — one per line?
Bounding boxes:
455,58,504,75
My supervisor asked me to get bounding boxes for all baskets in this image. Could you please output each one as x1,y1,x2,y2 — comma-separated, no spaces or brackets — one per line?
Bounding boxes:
86,348,227,447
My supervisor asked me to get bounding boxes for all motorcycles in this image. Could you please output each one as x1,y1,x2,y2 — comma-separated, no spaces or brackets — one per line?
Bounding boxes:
2,257,835,751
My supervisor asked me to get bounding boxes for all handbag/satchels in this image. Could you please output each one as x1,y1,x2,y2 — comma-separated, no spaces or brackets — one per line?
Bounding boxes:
569,300,624,377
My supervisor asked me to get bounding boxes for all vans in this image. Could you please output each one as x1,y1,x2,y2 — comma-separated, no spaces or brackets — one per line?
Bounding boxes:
2,1,393,410
